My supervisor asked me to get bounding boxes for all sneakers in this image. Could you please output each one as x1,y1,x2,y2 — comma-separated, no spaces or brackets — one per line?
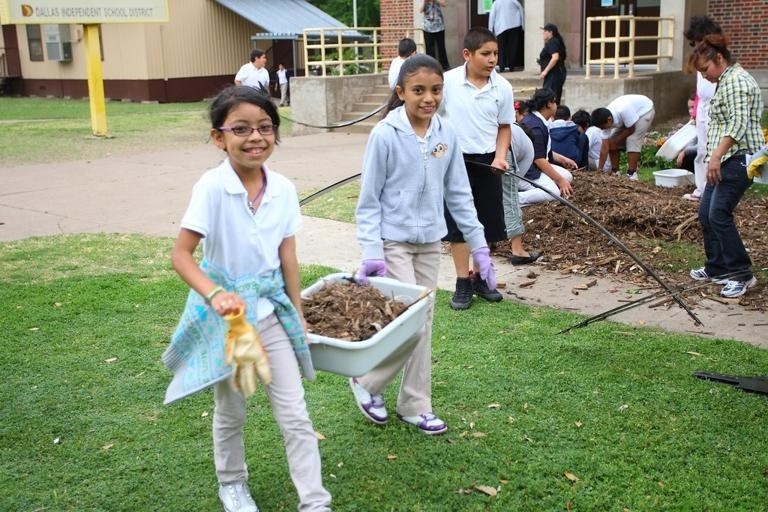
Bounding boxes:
473,271,503,302
396,412,448,435
626,172,638,181
218,479,260,512
683,193,701,201
348,376,389,426
450,271,476,309
720,275,758,298
610,170,622,178
690,266,729,285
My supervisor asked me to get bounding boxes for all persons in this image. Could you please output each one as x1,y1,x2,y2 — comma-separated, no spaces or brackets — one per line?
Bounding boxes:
274,63,290,107
687,38,764,300
535,23,567,106
487,1,525,72
591,94,656,180
679,17,730,203
514,91,578,209
386,37,415,84
233,48,268,94
502,125,543,266
548,106,613,171
440,29,515,310
676,94,700,171
170,83,340,512
348,55,502,435
417,1,453,71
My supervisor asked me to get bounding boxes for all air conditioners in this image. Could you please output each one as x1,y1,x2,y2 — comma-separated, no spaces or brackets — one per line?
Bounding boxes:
44,24,73,62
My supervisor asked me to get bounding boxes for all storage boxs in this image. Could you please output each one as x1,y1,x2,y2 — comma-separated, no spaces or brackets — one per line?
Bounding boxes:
653,168,693,188
656,120,696,161
300,272,435,377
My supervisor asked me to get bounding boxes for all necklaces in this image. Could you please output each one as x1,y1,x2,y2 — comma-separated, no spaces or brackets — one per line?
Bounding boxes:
244,178,265,213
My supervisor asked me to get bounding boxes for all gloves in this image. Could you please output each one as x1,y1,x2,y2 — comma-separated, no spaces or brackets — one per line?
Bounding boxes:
358,259,385,287
472,248,497,291
221,305,272,399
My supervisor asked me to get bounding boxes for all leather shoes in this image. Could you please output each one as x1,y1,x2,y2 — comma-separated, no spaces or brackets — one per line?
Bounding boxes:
511,250,544,265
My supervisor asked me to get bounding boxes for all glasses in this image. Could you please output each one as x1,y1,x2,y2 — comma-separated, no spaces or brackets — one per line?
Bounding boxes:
219,124,277,137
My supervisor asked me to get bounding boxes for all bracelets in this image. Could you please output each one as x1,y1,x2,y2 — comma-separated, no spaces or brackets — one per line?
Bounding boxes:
204,286,225,303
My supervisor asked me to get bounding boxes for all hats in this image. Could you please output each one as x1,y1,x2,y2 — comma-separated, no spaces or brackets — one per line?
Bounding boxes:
539,23,558,34
